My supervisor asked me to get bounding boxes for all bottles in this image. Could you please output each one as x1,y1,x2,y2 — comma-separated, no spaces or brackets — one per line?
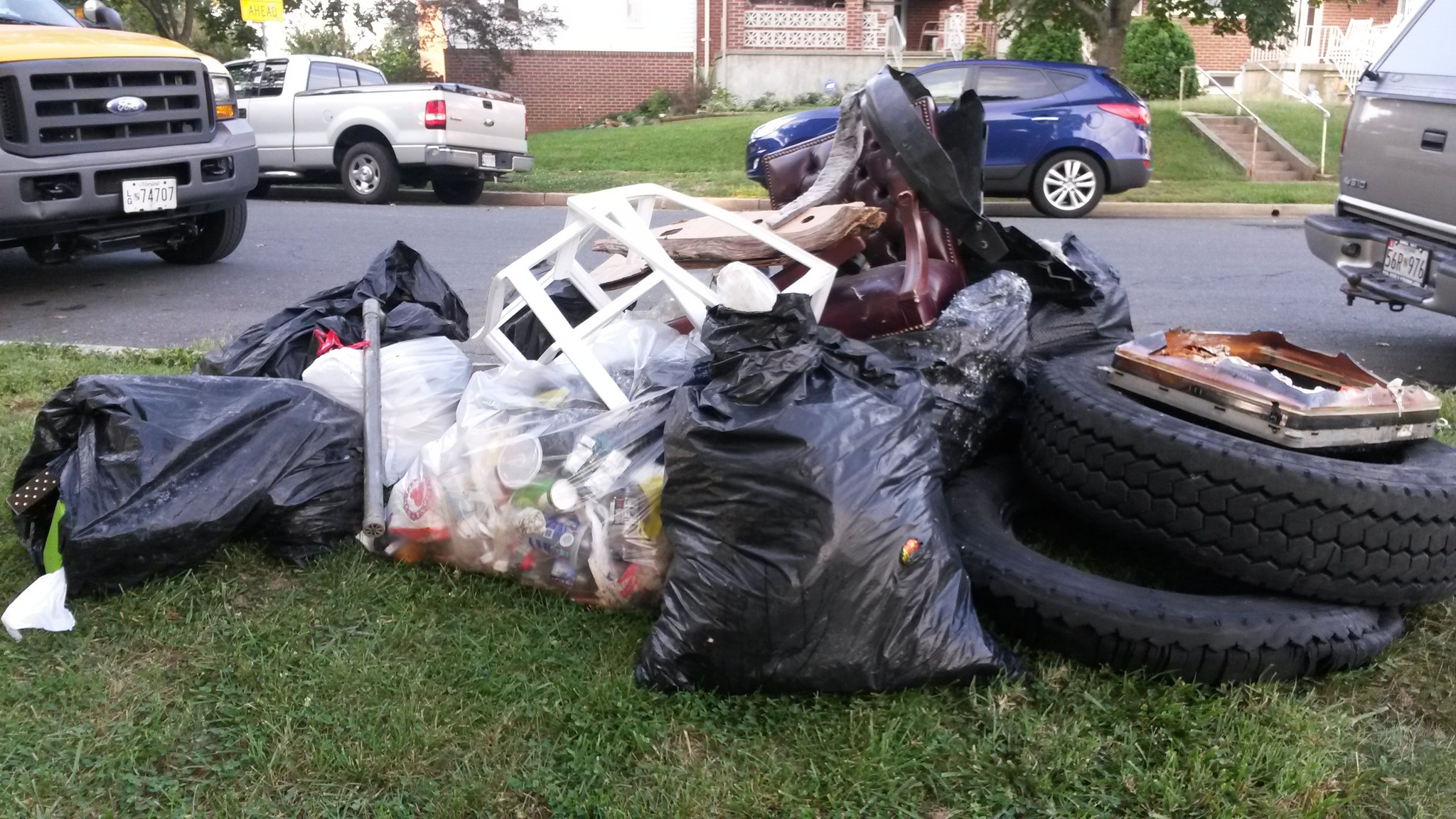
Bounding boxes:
422,443,498,565
520,557,599,594
607,487,642,557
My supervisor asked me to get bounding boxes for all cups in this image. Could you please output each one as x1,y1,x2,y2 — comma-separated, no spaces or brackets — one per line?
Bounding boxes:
539,478,579,514
528,515,591,568
494,434,542,489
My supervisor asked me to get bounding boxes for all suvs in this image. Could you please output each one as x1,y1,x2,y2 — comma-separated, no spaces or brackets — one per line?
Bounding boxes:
1300,0,1456,320
745,59,1155,219
0,0,261,265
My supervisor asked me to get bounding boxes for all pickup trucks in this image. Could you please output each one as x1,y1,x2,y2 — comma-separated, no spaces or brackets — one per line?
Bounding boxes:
223,54,534,206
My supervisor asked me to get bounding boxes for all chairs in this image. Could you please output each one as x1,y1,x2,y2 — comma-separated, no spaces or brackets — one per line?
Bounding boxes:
763,95,966,340
